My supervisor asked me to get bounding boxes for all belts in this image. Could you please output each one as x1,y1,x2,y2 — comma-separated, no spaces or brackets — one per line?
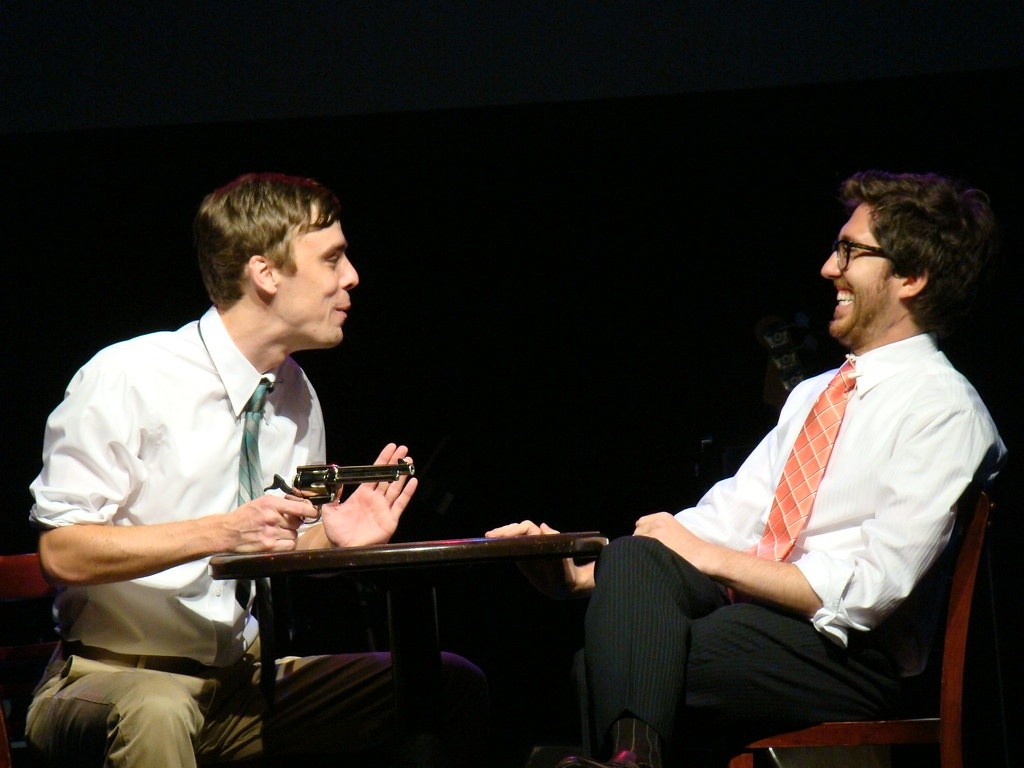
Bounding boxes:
62,638,256,691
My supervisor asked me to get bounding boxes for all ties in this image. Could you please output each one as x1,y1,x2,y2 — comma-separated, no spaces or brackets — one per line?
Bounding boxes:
727,363,857,606
234,380,275,717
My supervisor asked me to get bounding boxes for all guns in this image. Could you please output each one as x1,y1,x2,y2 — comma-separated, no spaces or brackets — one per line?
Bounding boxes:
261,456,417,527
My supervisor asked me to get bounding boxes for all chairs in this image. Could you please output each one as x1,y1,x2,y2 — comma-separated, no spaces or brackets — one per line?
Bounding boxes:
728,468,1010,768
0,552,92,768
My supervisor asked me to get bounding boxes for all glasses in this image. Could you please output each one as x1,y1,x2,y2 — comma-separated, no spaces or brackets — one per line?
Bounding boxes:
828,236,885,271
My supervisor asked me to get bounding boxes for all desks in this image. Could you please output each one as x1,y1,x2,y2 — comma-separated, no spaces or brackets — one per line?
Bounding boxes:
210,533,612,768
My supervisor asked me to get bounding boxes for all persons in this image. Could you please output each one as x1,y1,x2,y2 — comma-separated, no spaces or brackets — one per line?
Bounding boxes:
24,167,497,768
485,166,1006,768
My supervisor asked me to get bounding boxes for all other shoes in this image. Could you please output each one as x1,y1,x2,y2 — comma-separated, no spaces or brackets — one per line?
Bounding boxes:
555,749,640,768
